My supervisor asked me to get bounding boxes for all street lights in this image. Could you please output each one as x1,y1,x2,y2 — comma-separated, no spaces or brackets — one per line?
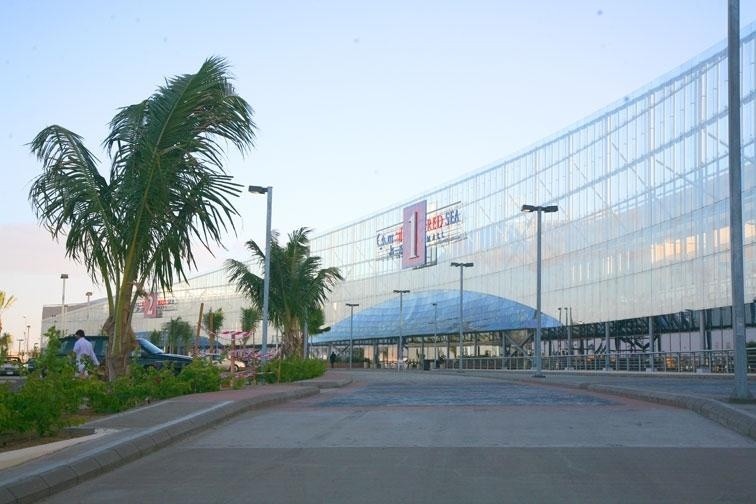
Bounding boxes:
59,272,69,315
27,325,29,353
17,338,23,352
392,289,411,361
450,260,476,369
433,302,438,361
520,203,559,380
86,291,93,307
247,184,275,378
346,303,361,368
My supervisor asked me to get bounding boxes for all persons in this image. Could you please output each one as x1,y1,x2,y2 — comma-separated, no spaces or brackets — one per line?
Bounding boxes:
73,328,93,377
330,351,336,368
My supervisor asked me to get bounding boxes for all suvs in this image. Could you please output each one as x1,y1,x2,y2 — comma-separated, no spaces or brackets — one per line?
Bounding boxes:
39,334,193,376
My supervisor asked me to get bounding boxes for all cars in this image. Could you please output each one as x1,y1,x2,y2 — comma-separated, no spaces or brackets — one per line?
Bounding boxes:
196,352,246,372
25,358,38,372
1,355,22,376
